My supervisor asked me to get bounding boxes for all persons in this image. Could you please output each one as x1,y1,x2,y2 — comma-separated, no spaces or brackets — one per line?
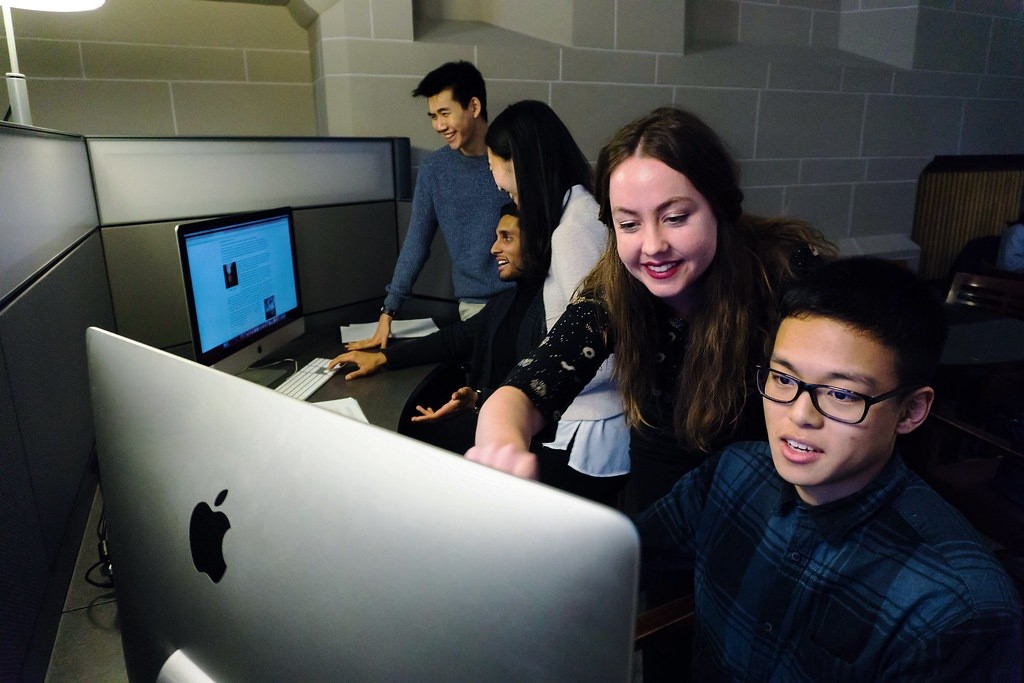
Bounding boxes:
632,254,1024,683
329,62,843,519
995,209,1024,272
266,299,276,320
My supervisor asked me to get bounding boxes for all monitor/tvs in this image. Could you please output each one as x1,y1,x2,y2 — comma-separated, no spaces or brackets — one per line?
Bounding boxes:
174,204,306,388
84,326,644,683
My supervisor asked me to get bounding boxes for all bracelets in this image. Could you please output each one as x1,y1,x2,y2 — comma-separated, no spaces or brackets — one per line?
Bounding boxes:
472,390,482,415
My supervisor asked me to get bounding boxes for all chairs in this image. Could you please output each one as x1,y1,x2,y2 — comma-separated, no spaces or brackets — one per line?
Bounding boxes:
631,270,1023,682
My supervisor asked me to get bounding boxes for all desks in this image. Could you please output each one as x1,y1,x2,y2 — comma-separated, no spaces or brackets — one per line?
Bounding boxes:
10,317,470,683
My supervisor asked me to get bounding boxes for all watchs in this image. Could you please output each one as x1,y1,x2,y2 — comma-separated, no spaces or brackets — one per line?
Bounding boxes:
378,303,396,318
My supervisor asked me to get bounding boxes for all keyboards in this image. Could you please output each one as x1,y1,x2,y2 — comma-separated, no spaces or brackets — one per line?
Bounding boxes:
273,357,348,401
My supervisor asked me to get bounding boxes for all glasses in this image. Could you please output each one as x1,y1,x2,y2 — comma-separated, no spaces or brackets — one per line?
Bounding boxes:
755,364,916,424
